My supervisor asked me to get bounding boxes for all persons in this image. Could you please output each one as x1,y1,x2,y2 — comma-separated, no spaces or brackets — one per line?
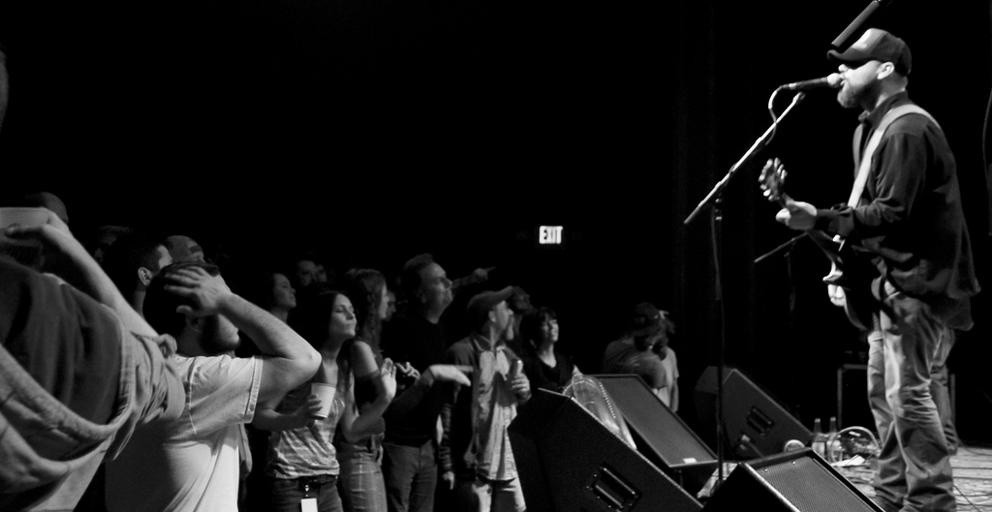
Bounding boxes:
775,26,978,512
930,295,974,458
3,190,579,510
604,303,679,414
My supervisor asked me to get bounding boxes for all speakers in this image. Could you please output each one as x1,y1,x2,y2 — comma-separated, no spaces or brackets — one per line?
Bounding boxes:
834,364,955,445
701,446,887,512
693,365,814,462
516,385,704,511
564,373,719,494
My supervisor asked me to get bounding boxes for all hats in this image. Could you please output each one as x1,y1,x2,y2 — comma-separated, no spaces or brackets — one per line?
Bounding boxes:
468,287,514,324
828,27,912,75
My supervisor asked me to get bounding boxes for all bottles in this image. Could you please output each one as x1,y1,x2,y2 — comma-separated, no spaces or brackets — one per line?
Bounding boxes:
811,417,824,458
825,416,841,459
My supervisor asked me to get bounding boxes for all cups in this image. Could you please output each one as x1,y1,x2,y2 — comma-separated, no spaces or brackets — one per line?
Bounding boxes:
308,382,336,419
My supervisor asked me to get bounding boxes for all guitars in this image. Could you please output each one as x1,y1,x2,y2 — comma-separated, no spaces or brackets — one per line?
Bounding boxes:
760,158,873,332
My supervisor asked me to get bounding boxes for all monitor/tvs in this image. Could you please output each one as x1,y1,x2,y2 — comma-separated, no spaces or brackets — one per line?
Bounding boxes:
540,225,563,245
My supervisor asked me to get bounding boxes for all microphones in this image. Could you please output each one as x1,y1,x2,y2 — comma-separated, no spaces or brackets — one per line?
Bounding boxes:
781,73,842,90
